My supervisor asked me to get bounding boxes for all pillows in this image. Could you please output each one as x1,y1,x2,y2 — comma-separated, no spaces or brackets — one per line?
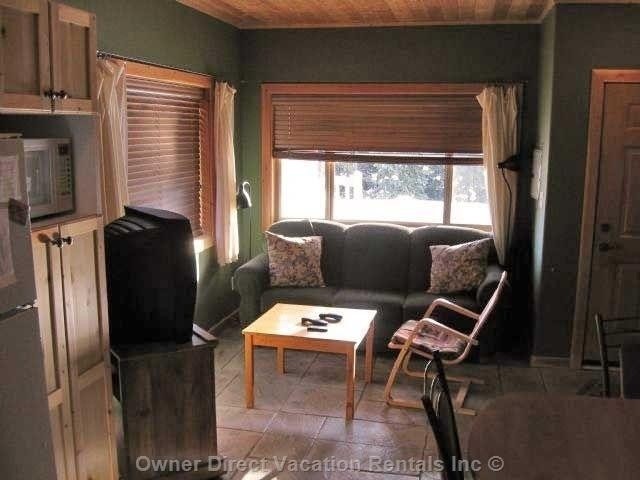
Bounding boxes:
426,238,492,295
264,229,326,288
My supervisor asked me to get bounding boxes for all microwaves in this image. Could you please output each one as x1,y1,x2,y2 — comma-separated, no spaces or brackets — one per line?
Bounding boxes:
24,137,73,219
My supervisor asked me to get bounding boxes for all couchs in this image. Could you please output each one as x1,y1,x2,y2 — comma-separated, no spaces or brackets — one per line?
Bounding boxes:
231,217,515,356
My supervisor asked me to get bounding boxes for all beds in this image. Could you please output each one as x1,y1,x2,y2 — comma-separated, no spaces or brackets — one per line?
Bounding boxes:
467,390,640,480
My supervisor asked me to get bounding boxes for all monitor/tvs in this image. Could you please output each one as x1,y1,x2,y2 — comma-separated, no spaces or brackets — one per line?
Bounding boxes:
103,205,197,349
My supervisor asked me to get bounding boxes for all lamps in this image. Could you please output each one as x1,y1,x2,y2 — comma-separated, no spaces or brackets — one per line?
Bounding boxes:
235,181,254,260
499,156,521,266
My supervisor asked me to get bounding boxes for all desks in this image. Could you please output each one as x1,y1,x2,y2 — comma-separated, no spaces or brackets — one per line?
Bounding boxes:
240,302,378,421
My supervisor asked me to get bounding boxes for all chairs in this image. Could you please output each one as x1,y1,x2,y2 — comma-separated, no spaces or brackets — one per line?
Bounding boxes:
420,348,465,480
595,311,640,398
384,271,508,417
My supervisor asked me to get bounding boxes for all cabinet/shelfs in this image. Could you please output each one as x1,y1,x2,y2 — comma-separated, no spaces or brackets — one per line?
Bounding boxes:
30,216,116,479
107,323,227,480
0,1,98,114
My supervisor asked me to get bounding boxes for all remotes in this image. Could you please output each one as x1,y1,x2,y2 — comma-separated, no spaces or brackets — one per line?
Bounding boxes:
307,328,328,332
320,312,342,323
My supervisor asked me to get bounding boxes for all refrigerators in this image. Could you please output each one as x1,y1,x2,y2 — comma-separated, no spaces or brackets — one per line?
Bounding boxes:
0,133,59,480
